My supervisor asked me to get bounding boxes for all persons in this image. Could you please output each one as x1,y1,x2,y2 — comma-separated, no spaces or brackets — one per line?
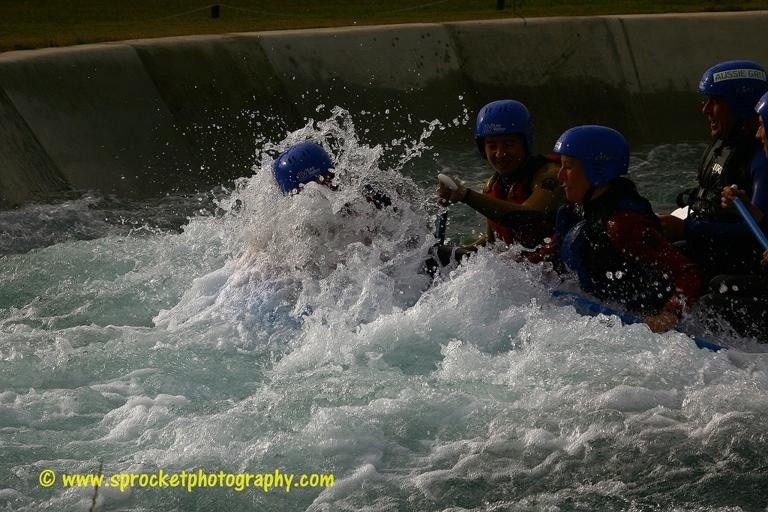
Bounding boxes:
272,141,397,221
434,100,570,275
656,58,768,277
551,125,688,335
708,90,766,348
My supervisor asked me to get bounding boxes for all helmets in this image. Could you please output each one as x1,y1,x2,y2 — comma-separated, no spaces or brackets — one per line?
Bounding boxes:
474,100,533,161
698,60,768,139
553,125,629,187
273,143,333,196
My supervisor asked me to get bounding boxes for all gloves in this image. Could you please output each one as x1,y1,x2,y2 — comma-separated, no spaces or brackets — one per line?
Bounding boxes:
760,249,768,265
721,186,764,225
437,171,465,206
645,311,680,334
